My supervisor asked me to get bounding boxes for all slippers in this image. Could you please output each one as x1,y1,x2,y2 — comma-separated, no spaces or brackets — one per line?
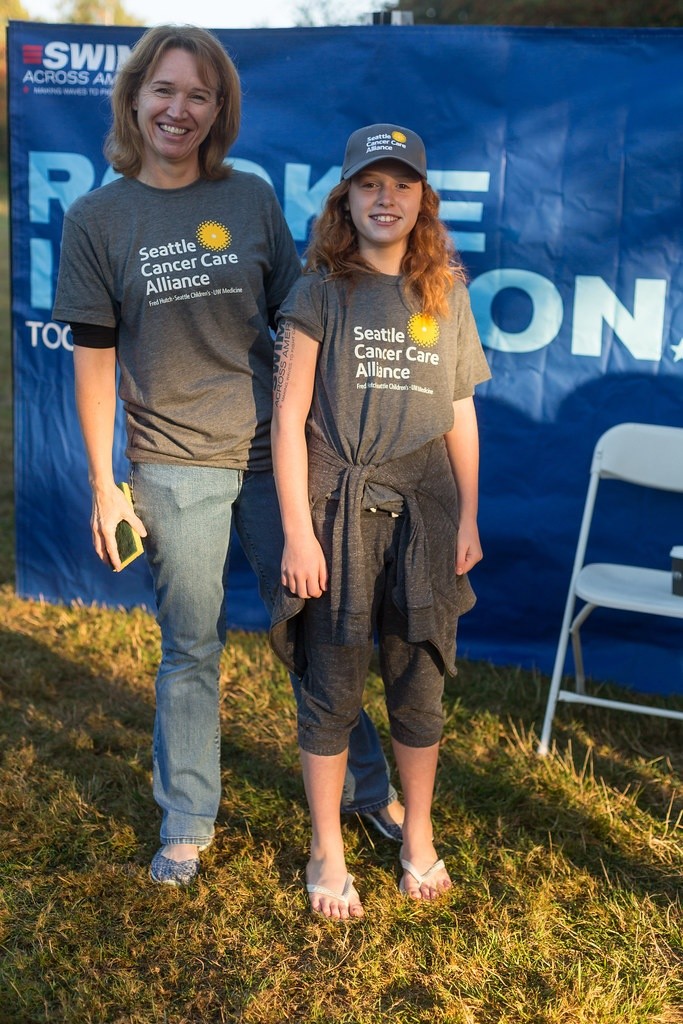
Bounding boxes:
400,844,454,902
305,873,362,921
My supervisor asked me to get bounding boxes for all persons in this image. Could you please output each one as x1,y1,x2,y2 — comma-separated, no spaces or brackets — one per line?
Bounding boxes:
49,22,407,885
270,122,493,922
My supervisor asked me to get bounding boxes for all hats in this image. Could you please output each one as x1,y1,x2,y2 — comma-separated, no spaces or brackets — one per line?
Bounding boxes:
339,123,428,181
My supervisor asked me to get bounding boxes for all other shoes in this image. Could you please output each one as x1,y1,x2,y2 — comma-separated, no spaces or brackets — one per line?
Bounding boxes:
149,844,201,886
365,811,403,841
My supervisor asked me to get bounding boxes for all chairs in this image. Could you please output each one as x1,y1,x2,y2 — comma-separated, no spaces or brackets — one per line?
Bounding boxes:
537,423,683,756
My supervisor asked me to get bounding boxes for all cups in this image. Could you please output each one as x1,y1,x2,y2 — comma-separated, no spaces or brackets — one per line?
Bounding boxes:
670,545,683,596
